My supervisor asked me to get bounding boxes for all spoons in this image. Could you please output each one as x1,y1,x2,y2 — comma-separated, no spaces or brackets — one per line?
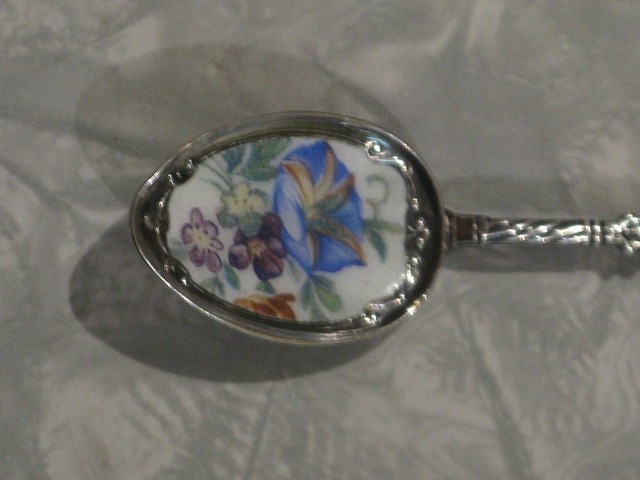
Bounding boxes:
127,108,640,347
